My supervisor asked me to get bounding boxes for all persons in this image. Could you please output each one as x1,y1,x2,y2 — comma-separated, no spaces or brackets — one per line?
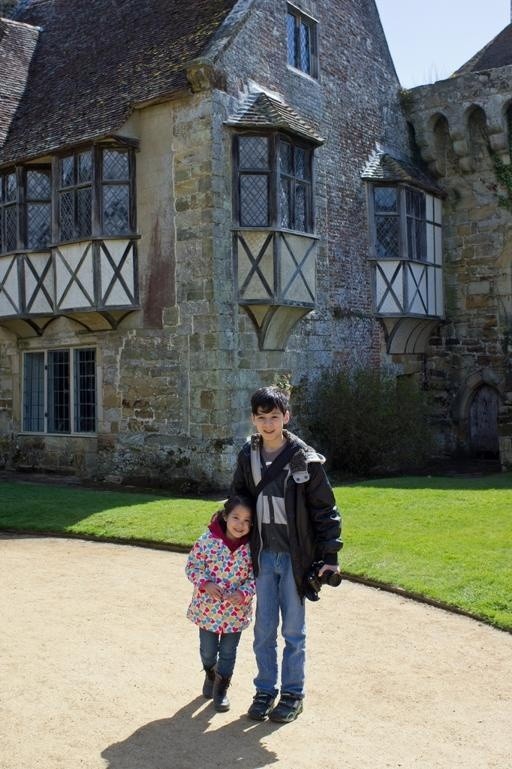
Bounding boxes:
185,495,256,711
228,386,343,723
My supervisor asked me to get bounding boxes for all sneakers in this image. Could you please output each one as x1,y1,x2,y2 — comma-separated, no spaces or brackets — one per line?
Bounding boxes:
269,692,304,723
248,690,275,721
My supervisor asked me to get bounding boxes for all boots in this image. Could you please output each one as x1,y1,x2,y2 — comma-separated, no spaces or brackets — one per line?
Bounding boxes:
212,670,232,712
203,663,217,699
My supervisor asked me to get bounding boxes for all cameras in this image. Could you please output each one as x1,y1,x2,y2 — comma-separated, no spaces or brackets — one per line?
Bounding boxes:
302,560,341,602
